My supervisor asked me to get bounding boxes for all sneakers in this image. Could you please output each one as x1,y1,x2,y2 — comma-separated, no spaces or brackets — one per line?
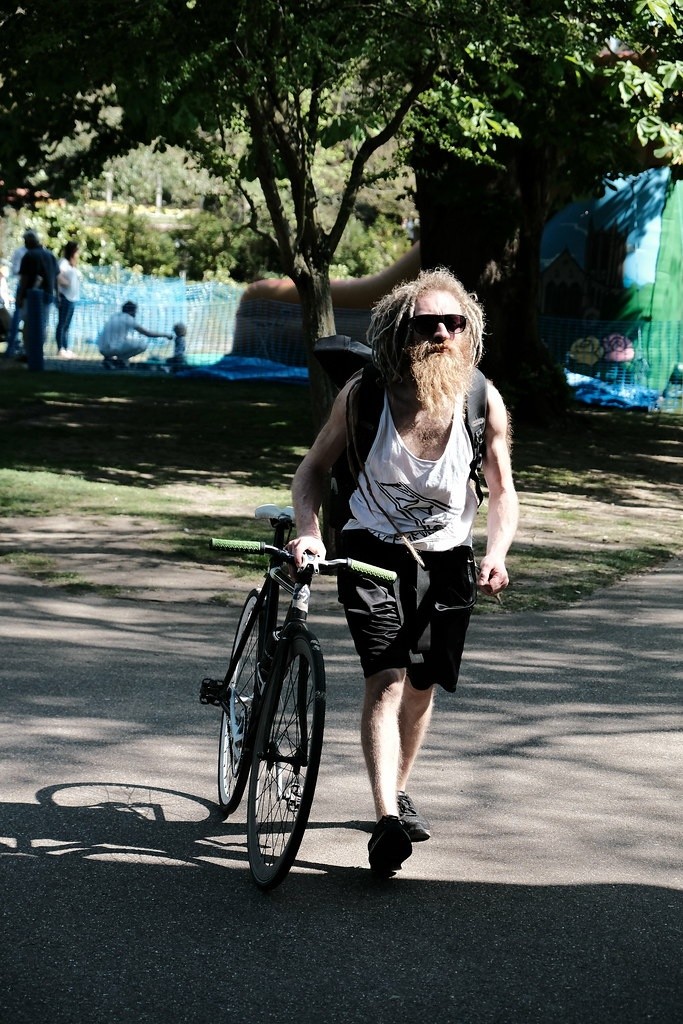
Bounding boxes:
366,815,412,873
396,792,431,840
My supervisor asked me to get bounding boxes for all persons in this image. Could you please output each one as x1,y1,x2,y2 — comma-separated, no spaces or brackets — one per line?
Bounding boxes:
287,272,519,870
99,302,173,368
6,231,81,371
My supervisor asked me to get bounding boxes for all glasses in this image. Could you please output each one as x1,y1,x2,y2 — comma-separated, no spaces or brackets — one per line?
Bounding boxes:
398,313,470,337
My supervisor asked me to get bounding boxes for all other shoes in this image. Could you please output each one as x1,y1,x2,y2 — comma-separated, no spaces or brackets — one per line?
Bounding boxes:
103,357,129,370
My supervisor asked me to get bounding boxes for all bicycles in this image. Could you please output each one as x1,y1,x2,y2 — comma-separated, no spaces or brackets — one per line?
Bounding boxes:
183,502,397,890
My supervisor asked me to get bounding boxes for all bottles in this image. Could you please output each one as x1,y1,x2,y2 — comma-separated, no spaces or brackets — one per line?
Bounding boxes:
262,630,281,673
